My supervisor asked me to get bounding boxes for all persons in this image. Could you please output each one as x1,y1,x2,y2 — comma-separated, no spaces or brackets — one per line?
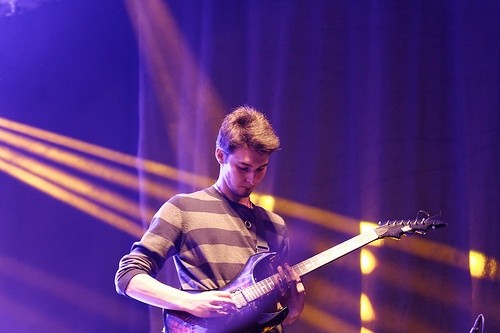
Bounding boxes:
114,106,307,333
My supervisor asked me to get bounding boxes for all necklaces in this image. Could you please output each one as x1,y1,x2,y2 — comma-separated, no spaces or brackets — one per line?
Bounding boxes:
213,181,253,229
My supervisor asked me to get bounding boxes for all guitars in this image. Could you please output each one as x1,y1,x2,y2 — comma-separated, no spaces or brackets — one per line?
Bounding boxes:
160,209,450,333
